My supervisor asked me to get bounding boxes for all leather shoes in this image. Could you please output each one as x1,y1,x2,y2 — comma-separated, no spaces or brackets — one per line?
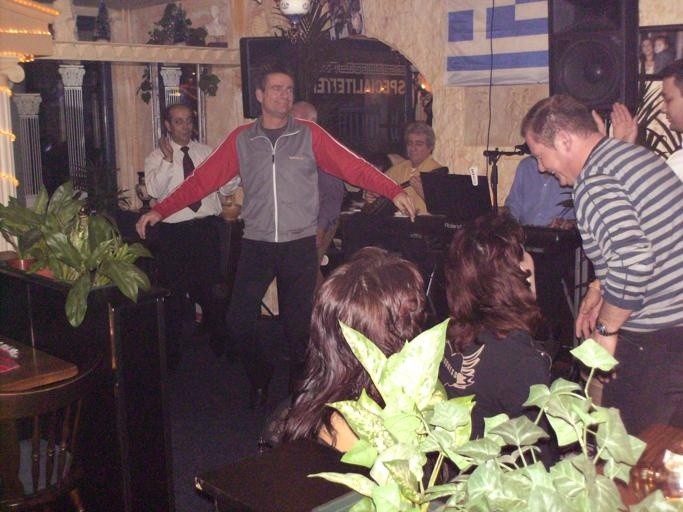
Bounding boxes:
249,363,276,410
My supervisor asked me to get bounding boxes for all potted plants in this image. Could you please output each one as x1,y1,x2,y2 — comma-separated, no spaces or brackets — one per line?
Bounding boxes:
135,1,219,105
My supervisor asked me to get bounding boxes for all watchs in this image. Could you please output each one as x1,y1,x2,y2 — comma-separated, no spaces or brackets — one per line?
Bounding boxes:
595,316,617,338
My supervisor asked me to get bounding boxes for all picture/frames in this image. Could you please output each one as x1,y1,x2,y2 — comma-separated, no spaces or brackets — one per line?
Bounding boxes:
639,25,683,81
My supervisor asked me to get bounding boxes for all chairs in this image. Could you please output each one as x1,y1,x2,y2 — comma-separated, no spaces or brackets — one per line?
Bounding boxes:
0,357,105,512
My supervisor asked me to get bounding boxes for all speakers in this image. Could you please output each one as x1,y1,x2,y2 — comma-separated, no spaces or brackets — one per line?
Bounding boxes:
547,0,640,122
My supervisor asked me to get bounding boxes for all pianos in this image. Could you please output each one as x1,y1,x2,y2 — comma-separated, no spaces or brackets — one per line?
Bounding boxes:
384,217,580,249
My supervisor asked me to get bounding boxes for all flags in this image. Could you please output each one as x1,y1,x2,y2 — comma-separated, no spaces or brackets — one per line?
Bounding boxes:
443,0,550,86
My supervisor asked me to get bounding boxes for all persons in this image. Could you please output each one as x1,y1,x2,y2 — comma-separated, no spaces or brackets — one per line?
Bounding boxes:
439,205,619,476
639,33,675,75
362,123,456,216
521,92,663,459
136,60,417,411
288,102,345,296
144,104,242,331
257,246,441,477
504,102,639,281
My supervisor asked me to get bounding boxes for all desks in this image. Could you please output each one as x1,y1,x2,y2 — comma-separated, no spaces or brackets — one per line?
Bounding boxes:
0,332,82,502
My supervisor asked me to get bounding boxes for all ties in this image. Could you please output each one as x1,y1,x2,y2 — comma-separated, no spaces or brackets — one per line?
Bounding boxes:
181,145,202,213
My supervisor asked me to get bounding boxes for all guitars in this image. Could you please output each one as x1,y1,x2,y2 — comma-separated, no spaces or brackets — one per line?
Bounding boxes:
314,161,457,281
